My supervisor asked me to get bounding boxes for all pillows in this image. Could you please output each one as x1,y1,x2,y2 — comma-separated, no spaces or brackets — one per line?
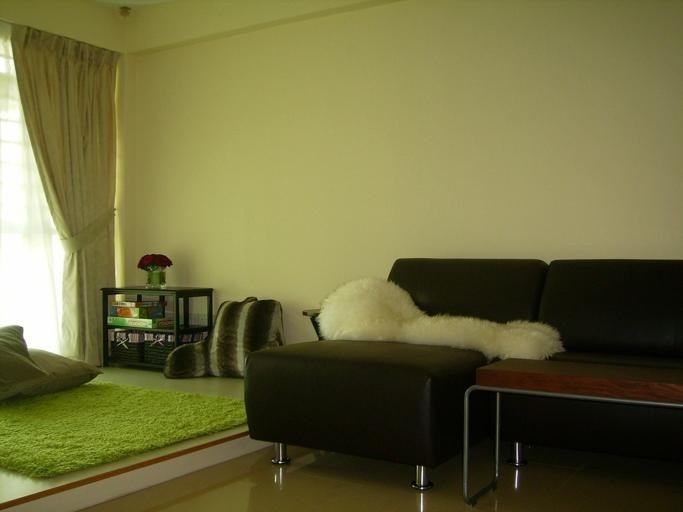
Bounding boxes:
21,349,105,397
0,326,51,401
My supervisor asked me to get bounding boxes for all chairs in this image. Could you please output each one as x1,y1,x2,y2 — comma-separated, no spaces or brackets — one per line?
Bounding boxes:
500,259,683,467
243,258,549,490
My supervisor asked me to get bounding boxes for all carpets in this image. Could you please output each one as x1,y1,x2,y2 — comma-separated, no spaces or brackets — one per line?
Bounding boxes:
0,380,248,478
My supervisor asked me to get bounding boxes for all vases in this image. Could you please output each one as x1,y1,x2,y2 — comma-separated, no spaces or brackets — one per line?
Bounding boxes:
146,271,166,289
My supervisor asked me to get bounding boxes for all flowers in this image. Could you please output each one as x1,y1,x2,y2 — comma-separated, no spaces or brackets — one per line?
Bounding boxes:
138,254,173,271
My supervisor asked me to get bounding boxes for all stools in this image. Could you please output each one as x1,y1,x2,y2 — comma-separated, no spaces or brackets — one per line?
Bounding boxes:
303,309,326,341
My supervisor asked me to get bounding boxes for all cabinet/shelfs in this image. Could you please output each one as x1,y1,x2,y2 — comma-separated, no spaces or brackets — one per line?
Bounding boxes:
101,285,213,369
